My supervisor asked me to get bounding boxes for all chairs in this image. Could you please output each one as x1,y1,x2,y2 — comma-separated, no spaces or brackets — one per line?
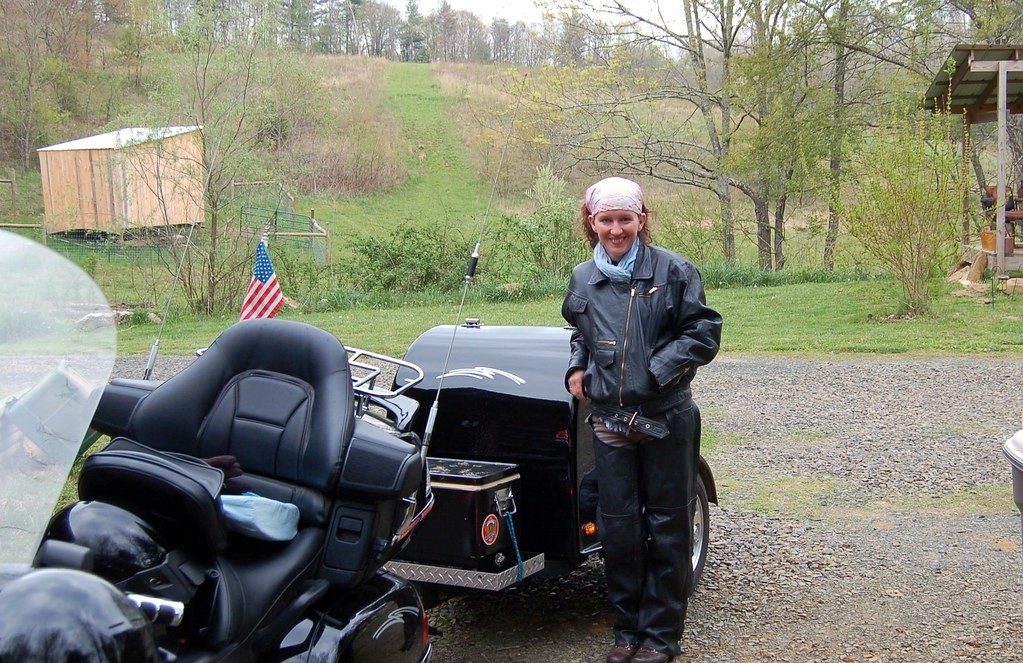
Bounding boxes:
981,186,1023,247
124,317,355,663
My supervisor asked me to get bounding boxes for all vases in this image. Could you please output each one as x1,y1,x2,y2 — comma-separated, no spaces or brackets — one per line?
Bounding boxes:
982,231,996,252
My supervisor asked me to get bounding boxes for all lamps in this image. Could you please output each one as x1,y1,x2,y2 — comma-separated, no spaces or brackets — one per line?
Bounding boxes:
997,274,1009,292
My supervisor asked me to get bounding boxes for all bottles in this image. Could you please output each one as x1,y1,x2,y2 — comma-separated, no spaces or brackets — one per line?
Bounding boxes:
126,593,185,628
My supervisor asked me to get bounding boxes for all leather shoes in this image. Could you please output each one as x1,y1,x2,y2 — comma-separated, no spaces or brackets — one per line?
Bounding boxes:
631,646,672,663
606,643,638,663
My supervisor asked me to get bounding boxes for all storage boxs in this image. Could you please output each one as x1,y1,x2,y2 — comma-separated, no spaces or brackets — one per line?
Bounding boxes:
397,456,521,574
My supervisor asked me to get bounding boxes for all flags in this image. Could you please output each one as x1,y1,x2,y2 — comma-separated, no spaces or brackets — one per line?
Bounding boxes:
241,243,286,319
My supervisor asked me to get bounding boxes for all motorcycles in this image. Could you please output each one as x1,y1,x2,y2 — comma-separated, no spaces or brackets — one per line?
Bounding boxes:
0,223,444,663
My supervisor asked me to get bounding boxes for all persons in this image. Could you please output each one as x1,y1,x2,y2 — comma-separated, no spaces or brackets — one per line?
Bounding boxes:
560,175,723,663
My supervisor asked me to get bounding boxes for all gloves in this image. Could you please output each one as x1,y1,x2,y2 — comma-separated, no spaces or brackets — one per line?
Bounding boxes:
204,456,241,488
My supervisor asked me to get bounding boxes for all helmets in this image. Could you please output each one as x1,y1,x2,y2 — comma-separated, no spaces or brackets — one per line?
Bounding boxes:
32,501,167,583
